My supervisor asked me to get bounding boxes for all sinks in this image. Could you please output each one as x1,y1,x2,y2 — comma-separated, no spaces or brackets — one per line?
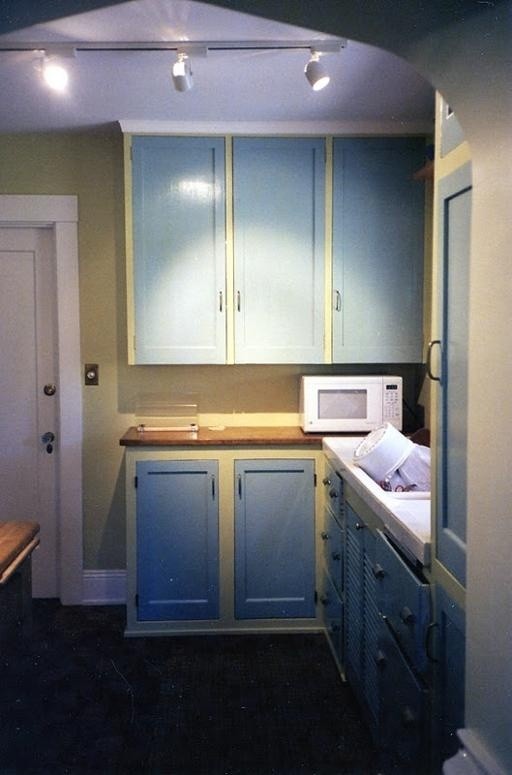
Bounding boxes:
350,462,431,500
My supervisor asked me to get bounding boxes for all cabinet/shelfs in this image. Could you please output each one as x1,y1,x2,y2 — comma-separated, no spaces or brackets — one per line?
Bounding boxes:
119,426,323,637
431,91,471,774
121,121,436,366
324,435,432,773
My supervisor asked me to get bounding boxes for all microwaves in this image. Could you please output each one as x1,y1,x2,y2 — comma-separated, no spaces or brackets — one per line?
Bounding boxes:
300,373,404,432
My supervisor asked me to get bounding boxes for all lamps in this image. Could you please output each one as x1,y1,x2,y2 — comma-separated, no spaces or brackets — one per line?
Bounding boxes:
306,52,329,94
40,59,71,95
172,60,194,93
